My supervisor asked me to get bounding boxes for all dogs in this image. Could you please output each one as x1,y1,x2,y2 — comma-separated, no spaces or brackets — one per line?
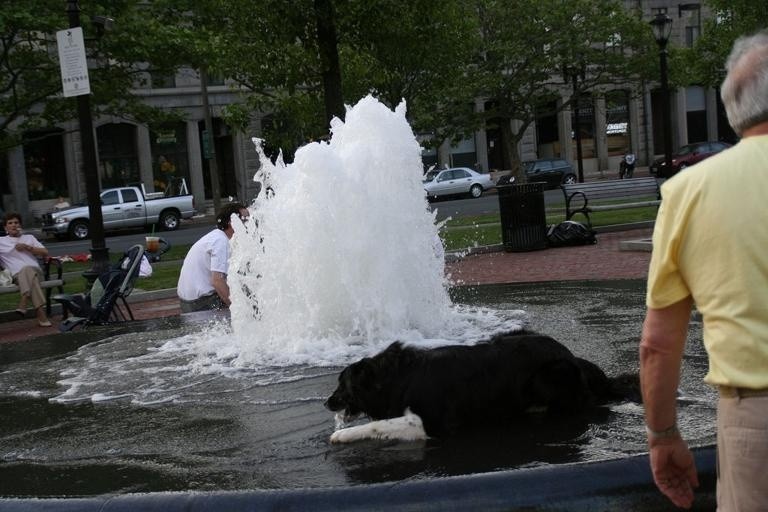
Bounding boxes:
323,327,644,447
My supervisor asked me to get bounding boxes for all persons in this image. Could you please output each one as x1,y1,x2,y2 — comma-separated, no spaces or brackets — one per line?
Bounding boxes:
638,28,768,512
52,196,71,212
0,213,52,330
175,205,251,314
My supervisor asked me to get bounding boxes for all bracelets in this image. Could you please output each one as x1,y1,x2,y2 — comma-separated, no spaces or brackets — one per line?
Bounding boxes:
642,423,676,440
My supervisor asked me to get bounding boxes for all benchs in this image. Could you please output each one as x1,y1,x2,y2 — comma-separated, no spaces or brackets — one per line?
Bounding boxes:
0,256,68,321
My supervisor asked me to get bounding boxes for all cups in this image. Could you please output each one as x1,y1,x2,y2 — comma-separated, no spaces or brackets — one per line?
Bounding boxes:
145,236,159,253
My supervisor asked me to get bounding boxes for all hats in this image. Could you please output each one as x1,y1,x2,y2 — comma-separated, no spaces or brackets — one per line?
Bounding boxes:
625,155,635,165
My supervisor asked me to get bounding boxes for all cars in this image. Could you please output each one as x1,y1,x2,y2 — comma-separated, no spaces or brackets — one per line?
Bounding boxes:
496,158,576,190
649,141,733,178
424,167,496,199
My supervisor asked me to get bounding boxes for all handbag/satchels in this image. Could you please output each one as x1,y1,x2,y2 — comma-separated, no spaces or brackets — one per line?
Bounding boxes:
1,269,14,288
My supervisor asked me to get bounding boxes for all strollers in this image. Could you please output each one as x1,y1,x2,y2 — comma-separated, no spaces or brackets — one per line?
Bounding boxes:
50,236,171,331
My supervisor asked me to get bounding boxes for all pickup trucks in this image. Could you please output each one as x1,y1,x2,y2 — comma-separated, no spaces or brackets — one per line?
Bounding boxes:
41,186,199,241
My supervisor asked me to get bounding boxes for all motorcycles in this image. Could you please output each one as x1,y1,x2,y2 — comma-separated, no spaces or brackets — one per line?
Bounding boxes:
619,154,638,179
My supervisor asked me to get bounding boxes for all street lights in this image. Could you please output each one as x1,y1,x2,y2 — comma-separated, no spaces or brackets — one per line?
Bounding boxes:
39,1,116,299
562,58,588,183
647,8,674,180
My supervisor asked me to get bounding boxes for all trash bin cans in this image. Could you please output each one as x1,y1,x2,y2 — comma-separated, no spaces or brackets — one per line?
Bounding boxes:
492,181,549,253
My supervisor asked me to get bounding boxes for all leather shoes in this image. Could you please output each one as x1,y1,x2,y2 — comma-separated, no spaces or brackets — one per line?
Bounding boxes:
36,316,51,327
15,308,26,316
